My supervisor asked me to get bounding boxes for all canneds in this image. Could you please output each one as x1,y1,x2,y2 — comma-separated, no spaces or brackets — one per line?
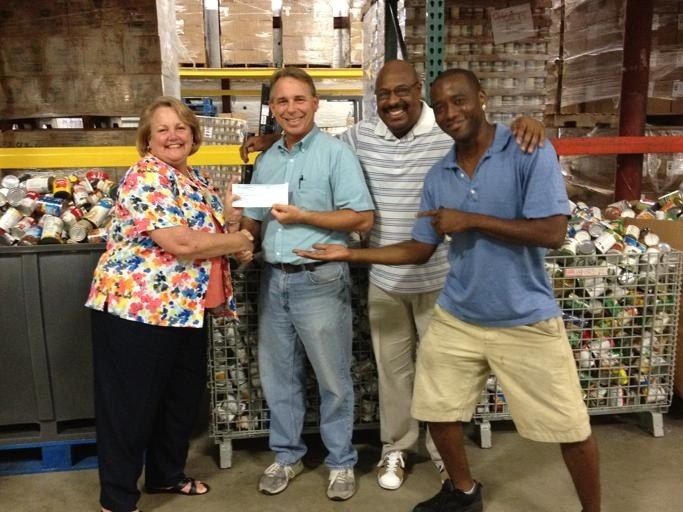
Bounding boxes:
0,170,119,245
475,187,683,414
210,255,377,430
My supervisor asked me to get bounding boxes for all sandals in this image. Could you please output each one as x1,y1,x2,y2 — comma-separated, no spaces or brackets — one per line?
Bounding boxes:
99,507,144,512
143,478,211,497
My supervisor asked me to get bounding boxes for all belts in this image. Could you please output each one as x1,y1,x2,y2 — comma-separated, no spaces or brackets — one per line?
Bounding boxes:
258,258,336,273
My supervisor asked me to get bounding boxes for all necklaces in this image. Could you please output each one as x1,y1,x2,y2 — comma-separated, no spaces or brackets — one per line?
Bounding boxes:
185,169,194,181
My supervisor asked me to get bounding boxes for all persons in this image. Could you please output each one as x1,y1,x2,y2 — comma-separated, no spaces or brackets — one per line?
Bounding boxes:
237,57,548,487
81,91,256,512
225,64,380,502
289,68,608,511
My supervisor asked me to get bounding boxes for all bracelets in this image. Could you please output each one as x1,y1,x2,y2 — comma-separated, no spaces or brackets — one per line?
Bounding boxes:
225,219,238,226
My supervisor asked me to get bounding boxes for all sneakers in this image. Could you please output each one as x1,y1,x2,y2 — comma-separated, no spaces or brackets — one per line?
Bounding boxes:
256,460,305,496
375,450,408,490
411,478,485,511
433,459,451,483
325,466,357,502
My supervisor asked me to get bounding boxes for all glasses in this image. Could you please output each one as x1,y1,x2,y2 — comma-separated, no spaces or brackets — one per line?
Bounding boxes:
372,83,420,100
